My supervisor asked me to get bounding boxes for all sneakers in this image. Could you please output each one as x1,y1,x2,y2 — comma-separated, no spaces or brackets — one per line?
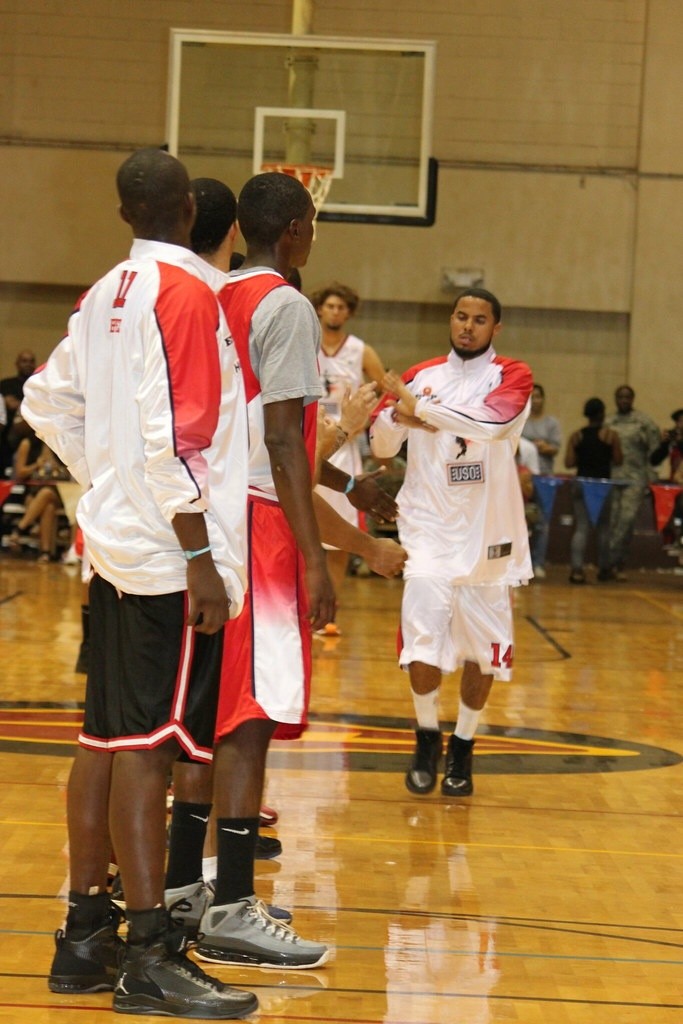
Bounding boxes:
112,899,257,1018
204,879,292,924
194,893,331,968
165,820,283,860
310,617,341,635
402,806,438,859
162,878,216,943
441,734,475,797
166,781,278,826
406,727,443,794
444,802,472,857
48,901,127,994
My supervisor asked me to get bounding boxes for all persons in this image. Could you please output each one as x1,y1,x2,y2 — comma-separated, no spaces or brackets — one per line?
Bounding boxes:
369,289,535,796
19,149,407,1018
357,383,683,586
0,348,70,564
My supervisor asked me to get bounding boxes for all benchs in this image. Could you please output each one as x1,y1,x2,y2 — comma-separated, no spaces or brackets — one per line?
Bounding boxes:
2,468,41,550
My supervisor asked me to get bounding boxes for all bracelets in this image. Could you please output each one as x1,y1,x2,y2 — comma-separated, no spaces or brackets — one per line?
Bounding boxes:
185,545,212,560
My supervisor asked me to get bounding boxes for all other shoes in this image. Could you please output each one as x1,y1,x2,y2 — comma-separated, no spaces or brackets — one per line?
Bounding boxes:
64,545,78,566
596,568,627,583
38,550,51,564
570,569,585,583
533,566,546,578
